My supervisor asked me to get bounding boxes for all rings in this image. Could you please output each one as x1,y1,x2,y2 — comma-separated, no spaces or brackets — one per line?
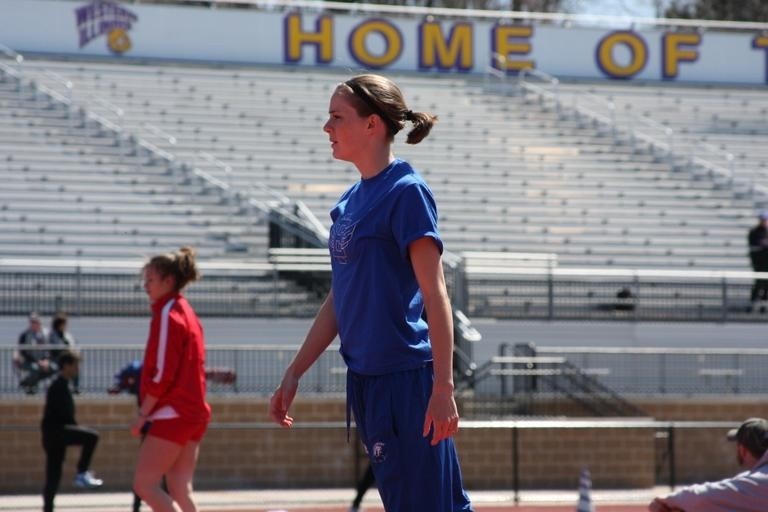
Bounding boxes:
448,428,455,433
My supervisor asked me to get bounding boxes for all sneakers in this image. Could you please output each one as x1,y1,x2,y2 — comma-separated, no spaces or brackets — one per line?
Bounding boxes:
75,471,104,488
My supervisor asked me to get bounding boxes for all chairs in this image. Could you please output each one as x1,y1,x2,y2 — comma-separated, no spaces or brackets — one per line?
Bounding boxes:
10,349,47,394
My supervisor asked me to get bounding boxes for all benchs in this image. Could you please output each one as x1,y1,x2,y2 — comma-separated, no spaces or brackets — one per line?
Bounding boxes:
0,55,768,310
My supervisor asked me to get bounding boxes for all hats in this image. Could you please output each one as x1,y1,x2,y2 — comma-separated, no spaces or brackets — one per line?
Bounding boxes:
726,418,768,448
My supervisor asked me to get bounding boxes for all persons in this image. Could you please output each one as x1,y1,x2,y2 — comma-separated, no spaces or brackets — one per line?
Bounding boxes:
46,310,82,395
11,310,60,394
265,72,477,512
129,243,213,512
37,351,105,512
106,357,171,512
645,416,767,511
742,211,768,314
349,463,377,512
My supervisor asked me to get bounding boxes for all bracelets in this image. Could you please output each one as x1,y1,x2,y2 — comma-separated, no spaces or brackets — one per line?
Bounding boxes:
137,411,148,419
